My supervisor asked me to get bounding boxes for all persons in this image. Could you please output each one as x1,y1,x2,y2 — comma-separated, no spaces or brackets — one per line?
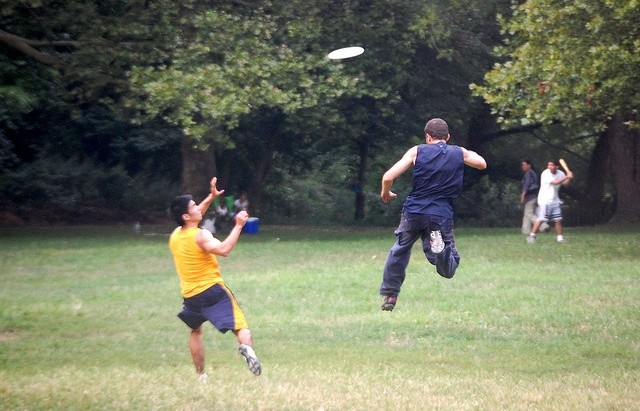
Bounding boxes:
234,190,250,218
379,117,485,310
528,159,573,244
215,197,231,230
167,175,263,385
519,158,550,235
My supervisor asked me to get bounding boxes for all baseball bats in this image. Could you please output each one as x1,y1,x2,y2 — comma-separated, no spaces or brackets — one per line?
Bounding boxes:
559,158,569,174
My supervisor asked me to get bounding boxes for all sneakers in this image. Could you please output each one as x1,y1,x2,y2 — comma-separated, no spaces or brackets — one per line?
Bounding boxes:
381,296,395,310
238,344,260,375
430,230,445,253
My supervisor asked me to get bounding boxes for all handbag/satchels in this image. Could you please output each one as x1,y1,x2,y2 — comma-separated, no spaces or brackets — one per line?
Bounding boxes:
520,171,539,194
